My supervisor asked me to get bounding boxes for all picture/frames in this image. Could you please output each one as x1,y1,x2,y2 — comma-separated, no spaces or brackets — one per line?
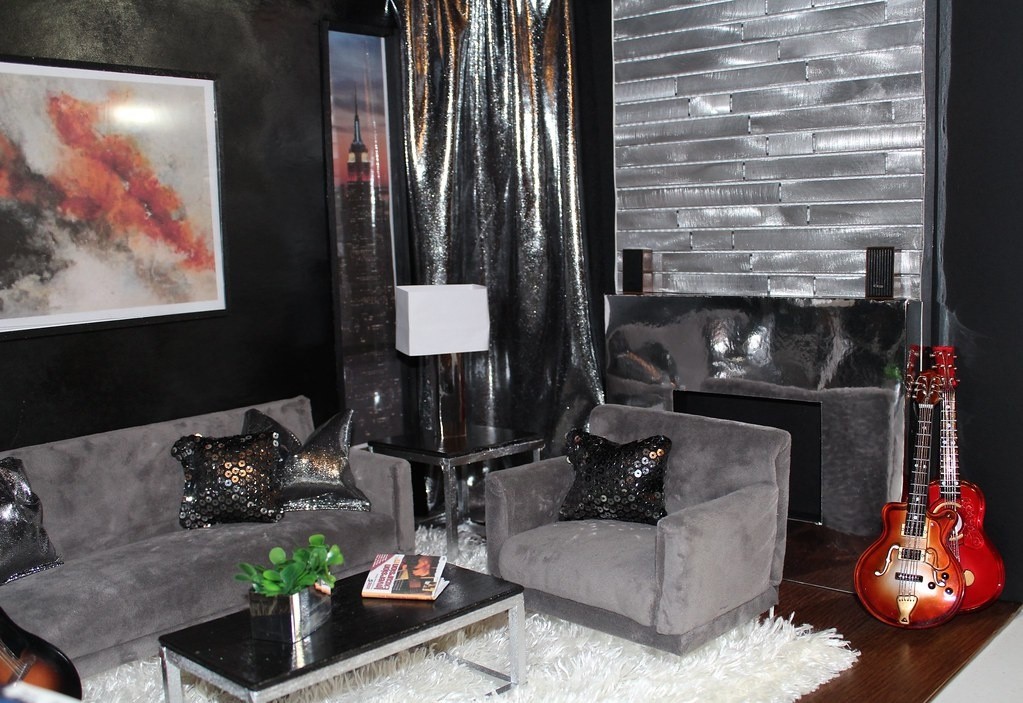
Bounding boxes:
323,20,408,452
0,51,239,341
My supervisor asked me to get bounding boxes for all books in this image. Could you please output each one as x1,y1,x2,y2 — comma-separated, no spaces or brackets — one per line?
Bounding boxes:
360,553,450,600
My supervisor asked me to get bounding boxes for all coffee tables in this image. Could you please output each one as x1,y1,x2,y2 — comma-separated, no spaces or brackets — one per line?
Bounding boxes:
158,555,528,703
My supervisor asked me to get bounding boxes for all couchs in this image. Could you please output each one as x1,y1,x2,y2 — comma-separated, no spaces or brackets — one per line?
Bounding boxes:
1,396,418,682
484,404,793,655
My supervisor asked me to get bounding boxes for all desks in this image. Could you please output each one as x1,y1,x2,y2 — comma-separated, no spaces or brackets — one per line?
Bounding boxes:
368,423,548,560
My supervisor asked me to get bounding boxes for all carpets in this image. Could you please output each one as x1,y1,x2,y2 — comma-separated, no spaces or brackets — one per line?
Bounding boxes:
81,525,860,702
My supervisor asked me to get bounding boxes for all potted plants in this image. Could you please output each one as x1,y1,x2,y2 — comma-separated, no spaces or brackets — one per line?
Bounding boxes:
234,532,345,646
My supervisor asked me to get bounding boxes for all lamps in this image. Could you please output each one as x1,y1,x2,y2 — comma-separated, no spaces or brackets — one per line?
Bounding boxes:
394,285,491,441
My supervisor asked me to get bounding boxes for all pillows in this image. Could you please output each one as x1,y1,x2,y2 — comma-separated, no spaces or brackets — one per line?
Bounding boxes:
0,457,64,586
241,407,370,512
171,426,290,532
557,429,672,526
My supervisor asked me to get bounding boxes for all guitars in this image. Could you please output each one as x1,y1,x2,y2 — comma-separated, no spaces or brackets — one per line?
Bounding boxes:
0,607,82,702
851,344,1004,631
